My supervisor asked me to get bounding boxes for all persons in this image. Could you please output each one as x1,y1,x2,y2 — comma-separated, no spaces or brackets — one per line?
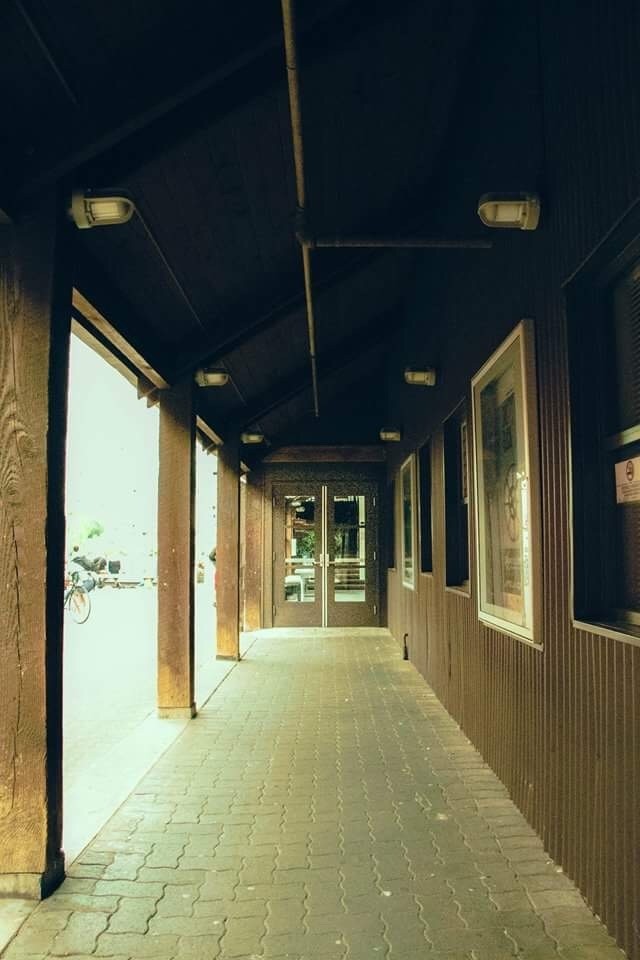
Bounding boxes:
71,545,79,583
210,547,217,565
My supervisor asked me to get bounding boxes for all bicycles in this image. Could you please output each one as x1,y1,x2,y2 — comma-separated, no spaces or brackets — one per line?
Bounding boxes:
63,571,92,624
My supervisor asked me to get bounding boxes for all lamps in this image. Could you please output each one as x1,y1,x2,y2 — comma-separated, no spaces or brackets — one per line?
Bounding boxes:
403,365,436,387
72,188,135,229
380,427,403,442
240,431,263,444
196,369,229,388
477,188,541,230
